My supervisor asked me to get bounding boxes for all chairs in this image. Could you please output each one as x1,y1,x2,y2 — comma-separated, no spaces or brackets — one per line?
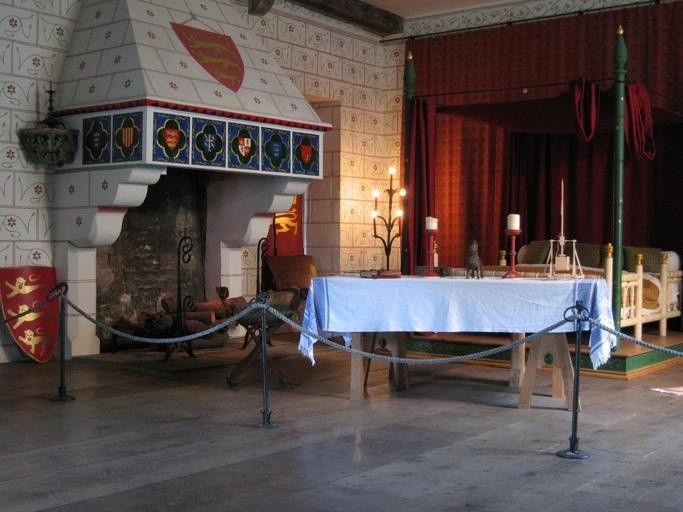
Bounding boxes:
226,283,309,389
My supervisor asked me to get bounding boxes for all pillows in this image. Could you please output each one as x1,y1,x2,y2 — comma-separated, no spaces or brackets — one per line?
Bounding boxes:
576,242,600,268
599,244,626,272
522,240,550,265
623,245,661,280
557,242,582,267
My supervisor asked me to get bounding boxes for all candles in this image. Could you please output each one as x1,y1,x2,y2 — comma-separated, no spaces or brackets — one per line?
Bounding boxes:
401,196,404,211
398,219,401,234
373,218,376,235
425,216,439,230
375,197,377,211
390,168,393,190
507,213,520,231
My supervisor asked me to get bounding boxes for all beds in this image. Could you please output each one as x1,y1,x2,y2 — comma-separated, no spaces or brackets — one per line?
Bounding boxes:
432,238,682,342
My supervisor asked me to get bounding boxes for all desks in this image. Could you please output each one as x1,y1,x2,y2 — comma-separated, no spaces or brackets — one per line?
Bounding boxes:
298,275,617,411
